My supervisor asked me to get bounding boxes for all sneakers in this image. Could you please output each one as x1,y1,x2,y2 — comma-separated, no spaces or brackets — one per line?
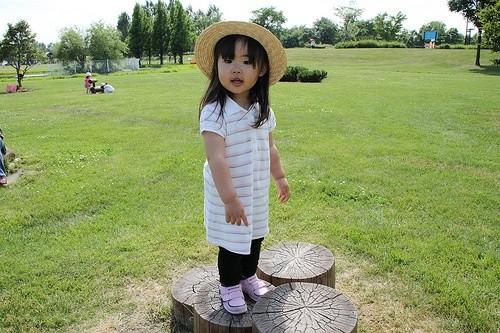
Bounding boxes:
240,272,268,300
219,282,247,313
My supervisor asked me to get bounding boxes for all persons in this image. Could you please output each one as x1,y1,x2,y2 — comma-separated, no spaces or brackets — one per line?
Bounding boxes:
430,41,435,49
310,39,316,48
195,20,291,316
100,83,114,93
84,72,92,94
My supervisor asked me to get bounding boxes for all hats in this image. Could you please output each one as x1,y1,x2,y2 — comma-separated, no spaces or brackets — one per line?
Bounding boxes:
194,20,287,86
